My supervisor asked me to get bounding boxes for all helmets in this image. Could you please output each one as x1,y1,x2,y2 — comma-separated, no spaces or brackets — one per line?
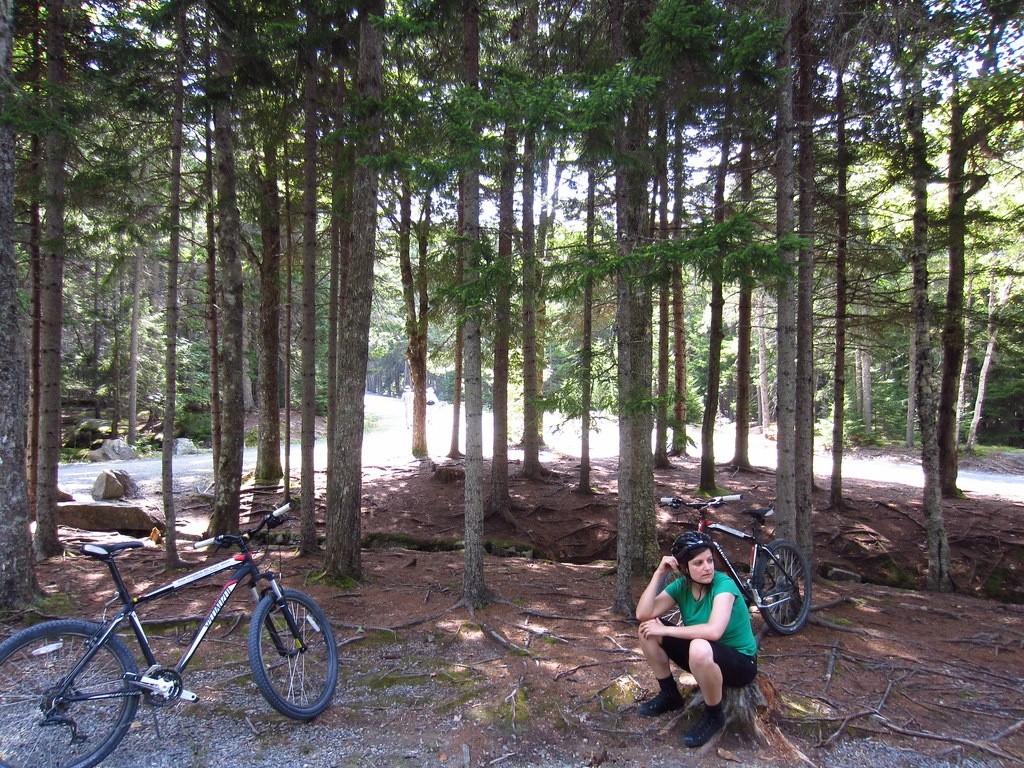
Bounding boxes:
671,532,715,562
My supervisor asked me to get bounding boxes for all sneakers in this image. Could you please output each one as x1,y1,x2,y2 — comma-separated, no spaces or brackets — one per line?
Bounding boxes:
682,708,726,747
635,691,685,716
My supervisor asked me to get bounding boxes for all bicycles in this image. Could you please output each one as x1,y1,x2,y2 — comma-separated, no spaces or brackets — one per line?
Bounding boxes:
659,495,813,637
0,502,339,768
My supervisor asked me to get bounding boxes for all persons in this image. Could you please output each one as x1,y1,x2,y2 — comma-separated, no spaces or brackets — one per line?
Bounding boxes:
635,530,757,746
400,385,438,431
